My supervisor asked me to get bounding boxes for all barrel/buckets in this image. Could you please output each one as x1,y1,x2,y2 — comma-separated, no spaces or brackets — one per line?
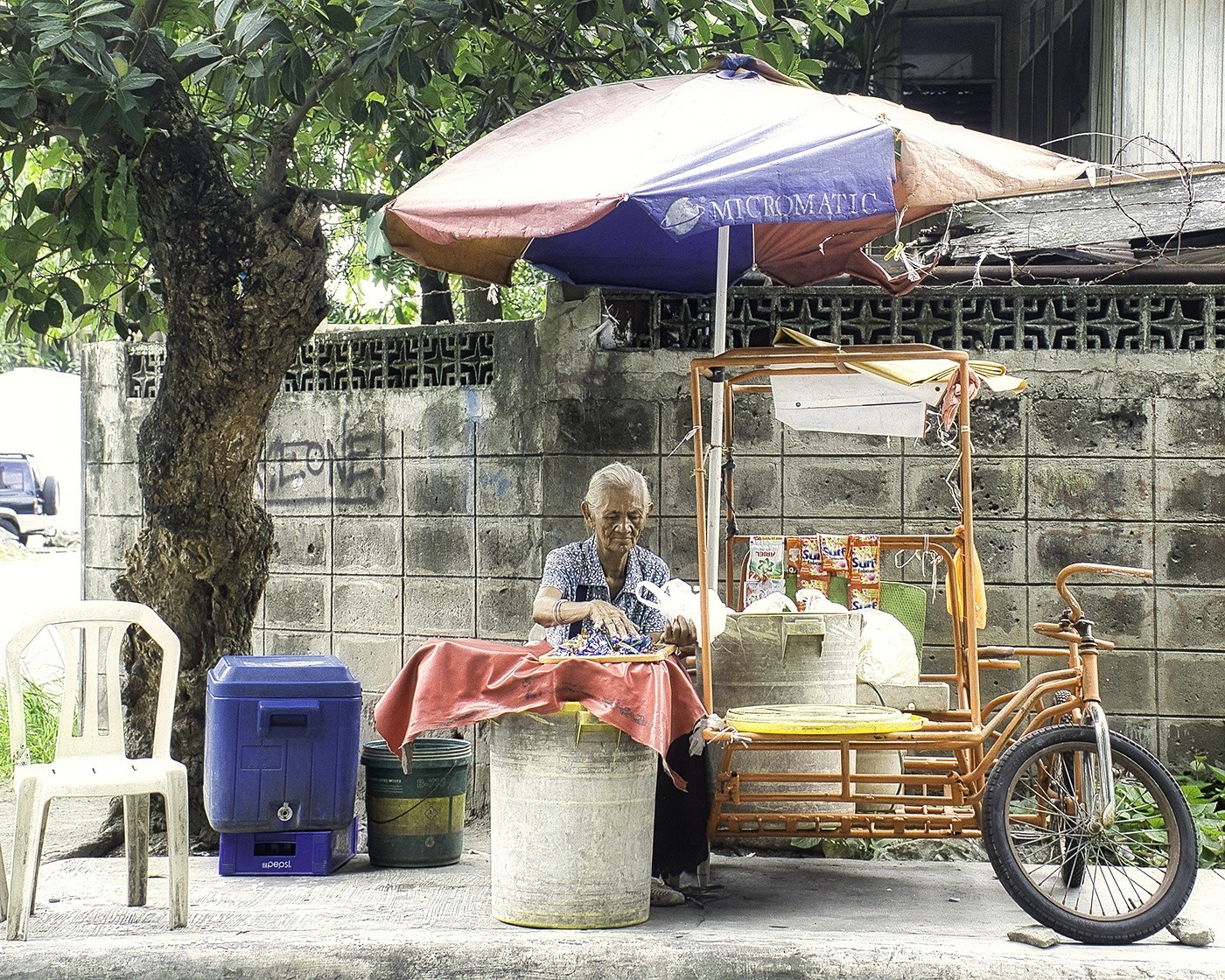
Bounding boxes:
853,748,908,813
694,613,860,841
359,737,473,868
483,697,662,928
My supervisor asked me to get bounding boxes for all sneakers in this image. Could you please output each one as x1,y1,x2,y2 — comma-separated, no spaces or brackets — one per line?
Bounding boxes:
650,873,684,906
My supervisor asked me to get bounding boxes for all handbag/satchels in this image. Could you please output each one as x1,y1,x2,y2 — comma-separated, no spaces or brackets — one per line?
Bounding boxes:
946,524,987,630
636,579,727,648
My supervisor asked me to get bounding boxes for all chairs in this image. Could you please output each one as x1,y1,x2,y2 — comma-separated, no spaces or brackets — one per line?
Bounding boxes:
5,600,188,941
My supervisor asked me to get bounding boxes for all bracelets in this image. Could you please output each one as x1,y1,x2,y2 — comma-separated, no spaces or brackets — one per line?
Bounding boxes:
552,600,568,626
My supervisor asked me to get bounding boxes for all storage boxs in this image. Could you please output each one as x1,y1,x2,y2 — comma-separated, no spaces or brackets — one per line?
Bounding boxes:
856,682,950,711
219,814,358,876
203,655,362,833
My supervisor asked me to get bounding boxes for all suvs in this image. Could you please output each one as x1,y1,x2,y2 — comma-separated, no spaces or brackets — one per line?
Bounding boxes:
0,452,61,547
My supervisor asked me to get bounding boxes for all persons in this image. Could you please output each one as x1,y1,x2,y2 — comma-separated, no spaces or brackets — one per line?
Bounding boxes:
534,459,714,906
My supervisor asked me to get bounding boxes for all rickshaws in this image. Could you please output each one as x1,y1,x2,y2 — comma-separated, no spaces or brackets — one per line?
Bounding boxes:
685,341,1201,951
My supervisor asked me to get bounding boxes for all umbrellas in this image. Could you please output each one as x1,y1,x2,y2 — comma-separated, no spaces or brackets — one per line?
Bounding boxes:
364,68,1097,589
